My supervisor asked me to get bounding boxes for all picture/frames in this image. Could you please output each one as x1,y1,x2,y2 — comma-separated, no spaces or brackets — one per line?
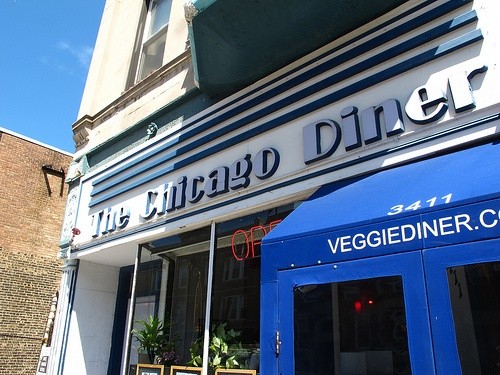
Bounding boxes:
136,363,164,375
215,369,256,375
170,366,203,375
